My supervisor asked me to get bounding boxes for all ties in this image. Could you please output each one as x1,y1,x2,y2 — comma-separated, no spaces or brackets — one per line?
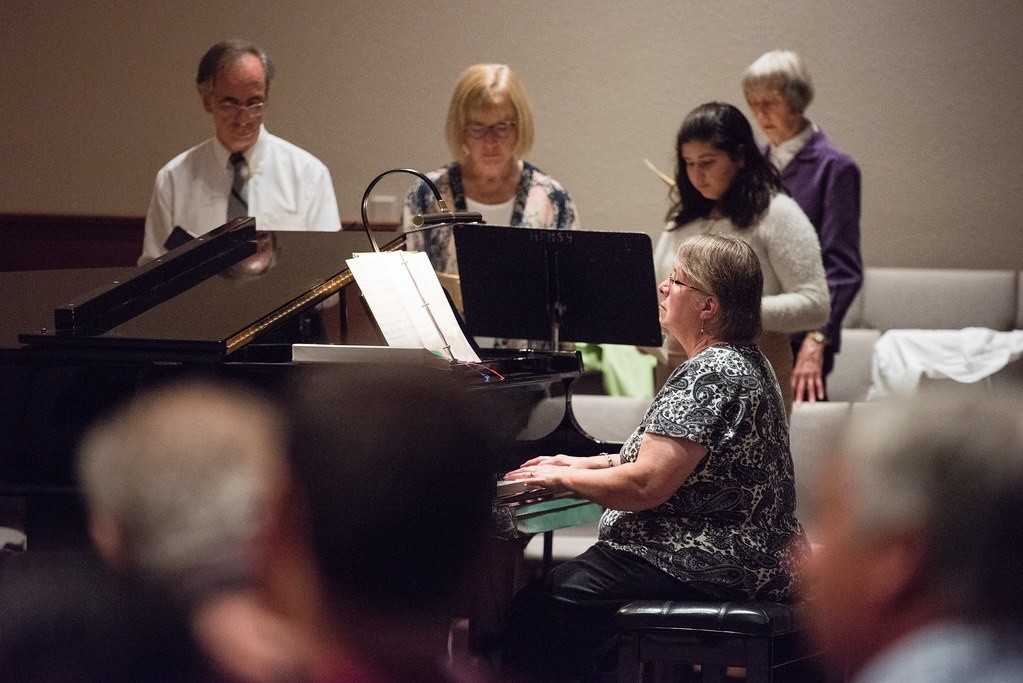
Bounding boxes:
227,150,250,220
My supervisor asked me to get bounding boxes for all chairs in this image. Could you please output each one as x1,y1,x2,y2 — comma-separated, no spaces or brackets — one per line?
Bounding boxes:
519,269,1023,581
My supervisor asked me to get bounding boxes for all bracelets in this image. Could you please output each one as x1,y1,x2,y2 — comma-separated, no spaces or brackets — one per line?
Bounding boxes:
600,451,613,468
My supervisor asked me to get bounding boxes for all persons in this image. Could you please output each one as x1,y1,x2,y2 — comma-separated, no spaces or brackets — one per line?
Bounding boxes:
493,231,803,683
742,49,863,404
781,378,1022,683
138,39,342,343
626,101,833,426
0,381,366,683
400,60,574,351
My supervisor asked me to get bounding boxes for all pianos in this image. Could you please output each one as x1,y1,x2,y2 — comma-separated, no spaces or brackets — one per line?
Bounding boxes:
0,216,624,683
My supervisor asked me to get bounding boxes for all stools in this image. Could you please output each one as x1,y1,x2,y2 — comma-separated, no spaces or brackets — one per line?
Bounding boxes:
616,599,824,683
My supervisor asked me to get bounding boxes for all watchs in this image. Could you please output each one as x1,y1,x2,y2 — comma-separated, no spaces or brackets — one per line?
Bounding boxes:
806,330,828,346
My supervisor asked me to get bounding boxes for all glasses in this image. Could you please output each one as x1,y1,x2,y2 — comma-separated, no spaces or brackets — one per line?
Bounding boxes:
464,121,520,139
205,90,270,117
668,272,713,298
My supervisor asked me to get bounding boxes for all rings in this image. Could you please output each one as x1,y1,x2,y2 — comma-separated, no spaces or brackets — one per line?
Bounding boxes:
531,472,535,479
